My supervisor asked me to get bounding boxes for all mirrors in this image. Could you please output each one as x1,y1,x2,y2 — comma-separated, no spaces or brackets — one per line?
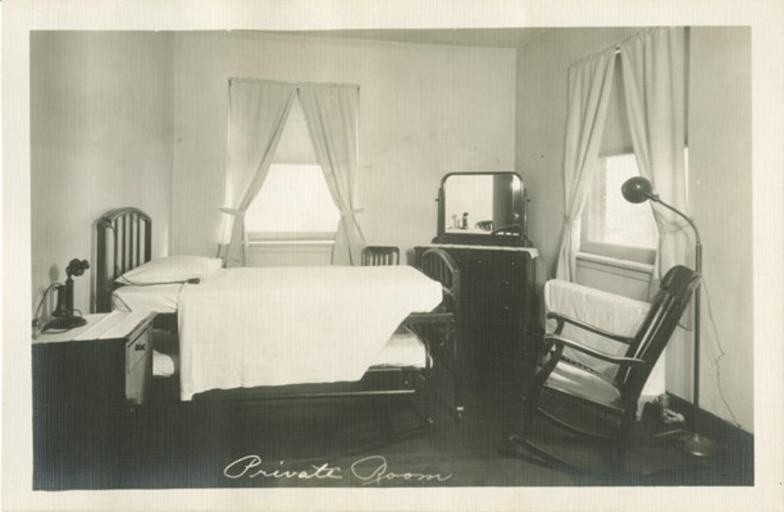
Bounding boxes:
437,170,531,246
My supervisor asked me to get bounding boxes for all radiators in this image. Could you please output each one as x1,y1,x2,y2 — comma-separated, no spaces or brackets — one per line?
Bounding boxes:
541,279,665,414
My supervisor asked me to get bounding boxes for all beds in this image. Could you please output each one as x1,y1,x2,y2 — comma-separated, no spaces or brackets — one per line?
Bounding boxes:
94,206,463,438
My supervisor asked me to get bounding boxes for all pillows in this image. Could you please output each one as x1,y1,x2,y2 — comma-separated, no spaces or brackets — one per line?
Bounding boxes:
115,254,224,286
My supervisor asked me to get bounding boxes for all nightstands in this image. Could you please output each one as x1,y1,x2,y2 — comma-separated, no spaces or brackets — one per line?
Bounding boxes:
37,308,164,441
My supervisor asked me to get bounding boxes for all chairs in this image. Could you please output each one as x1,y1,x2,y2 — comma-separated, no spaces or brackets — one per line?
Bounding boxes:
504,265,699,478
360,244,401,269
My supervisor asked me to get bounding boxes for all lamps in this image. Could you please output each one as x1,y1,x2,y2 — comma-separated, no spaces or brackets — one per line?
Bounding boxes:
620,175,715,459
